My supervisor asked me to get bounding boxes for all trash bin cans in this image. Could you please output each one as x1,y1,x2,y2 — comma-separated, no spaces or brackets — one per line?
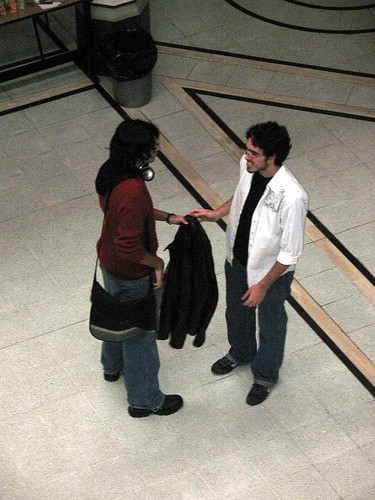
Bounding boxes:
100,29,155,108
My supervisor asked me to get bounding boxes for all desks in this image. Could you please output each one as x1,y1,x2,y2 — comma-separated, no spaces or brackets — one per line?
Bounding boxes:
0,0,99,83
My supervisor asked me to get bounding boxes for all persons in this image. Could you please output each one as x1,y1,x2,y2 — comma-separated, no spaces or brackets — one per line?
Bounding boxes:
189,121,309,405
95,119,189,419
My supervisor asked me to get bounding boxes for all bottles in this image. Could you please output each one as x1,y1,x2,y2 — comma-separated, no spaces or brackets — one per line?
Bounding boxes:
0,0,25,16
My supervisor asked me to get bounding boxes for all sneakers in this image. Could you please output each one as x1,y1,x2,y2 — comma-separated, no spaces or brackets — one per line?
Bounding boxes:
212,357,237,374
104,372,118,382
245,384,270,406
128,395,184,417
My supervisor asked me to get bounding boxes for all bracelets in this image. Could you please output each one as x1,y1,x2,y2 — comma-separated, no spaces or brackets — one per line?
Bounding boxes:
166,213,175,224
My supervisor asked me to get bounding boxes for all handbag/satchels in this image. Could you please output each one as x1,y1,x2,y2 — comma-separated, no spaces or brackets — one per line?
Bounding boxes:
88,280,157,342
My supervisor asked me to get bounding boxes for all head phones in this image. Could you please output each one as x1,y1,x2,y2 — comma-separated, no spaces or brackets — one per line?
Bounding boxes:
136,163,154,181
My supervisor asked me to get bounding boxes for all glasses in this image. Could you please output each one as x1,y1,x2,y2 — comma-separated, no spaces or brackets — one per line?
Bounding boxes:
242,145,275,159
